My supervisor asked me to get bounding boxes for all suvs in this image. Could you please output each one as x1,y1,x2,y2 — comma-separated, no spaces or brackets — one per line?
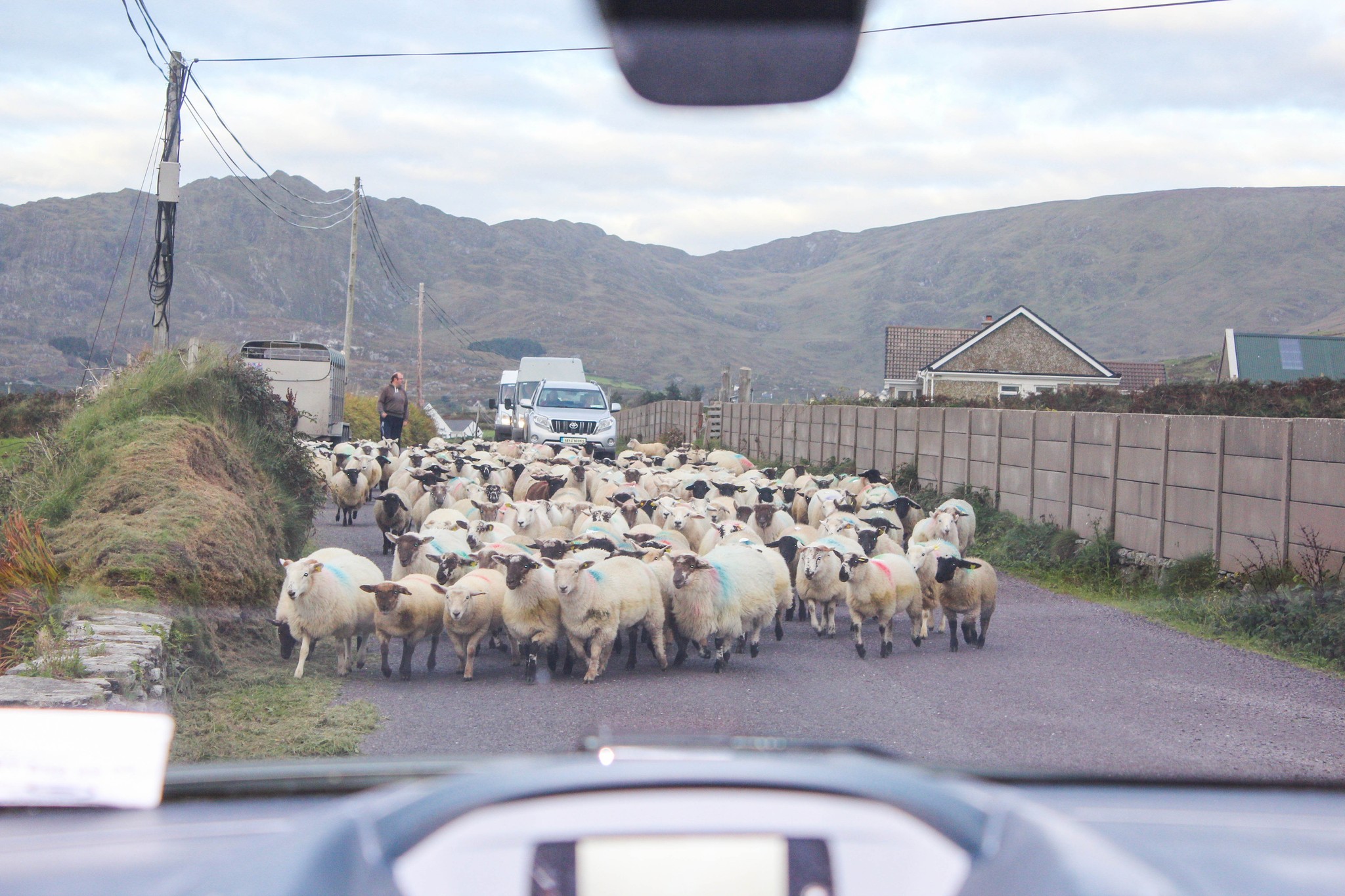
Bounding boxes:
520,378,622,461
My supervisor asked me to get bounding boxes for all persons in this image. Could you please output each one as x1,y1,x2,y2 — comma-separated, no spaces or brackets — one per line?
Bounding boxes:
577,394,591,408
378,372,408,452
544,391,561,405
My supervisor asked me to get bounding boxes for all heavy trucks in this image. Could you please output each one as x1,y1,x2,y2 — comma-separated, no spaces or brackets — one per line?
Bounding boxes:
238,340,353,451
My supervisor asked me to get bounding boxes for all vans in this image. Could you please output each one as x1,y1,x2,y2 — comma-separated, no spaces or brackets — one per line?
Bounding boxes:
505,357,586,443
489,370,518,442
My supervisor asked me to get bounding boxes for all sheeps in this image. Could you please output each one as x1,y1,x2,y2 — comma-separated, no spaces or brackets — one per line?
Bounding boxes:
273,438,1000,685
